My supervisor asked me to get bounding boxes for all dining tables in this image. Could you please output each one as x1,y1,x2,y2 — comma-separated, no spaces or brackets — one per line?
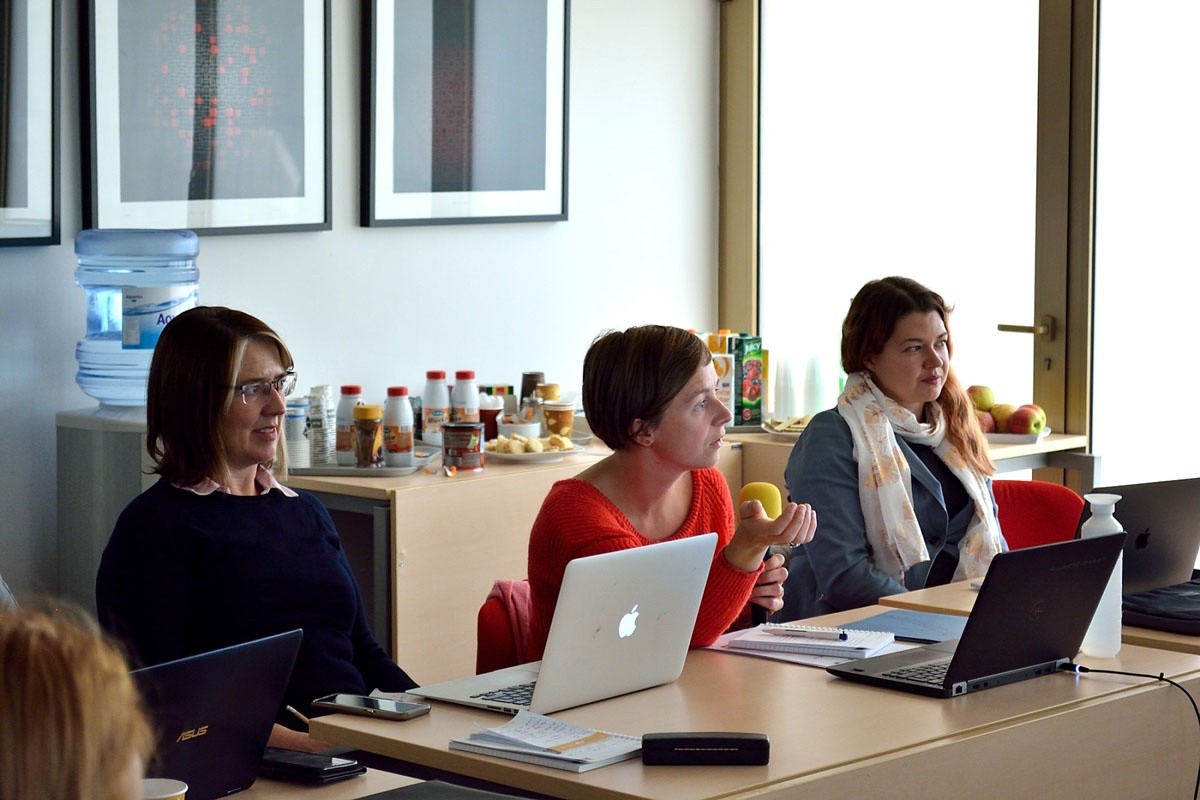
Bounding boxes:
266,417,743,688
720,422,1087,533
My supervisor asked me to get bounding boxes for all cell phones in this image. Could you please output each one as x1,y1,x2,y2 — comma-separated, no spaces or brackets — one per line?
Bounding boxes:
257,745,363,775
311,692,432,721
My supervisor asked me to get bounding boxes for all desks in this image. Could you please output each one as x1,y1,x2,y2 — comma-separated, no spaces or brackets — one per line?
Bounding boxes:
877,576,1199,656
213,762,425,800
308,604,1200,800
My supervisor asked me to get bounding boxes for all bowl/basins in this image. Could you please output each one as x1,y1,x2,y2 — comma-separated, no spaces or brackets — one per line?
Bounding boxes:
480,409,541,438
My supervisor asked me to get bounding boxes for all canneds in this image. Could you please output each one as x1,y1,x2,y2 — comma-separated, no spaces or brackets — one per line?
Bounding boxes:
440,422,486,471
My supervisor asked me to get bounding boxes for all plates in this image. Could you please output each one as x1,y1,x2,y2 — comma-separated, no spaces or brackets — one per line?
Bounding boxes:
481,444,585,464
762,423,802,442
984,427,1052,444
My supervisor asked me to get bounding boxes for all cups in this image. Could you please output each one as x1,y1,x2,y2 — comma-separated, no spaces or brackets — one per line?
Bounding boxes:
285,385,337,468
141,777,189,800
520,373,575,438
441,421,485,475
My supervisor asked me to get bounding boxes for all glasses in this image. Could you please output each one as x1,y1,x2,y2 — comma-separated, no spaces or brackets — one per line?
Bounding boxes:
233,371,297,406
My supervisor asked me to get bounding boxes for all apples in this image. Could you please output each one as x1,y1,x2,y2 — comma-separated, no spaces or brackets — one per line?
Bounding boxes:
967,385,1046,434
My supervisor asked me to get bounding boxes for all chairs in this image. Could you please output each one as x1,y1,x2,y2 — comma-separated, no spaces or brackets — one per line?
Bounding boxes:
991,480,1086,553
475,579,530,677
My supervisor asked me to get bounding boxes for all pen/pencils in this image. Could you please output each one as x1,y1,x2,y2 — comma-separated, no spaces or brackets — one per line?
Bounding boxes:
287,705,310,725
761,628,847,641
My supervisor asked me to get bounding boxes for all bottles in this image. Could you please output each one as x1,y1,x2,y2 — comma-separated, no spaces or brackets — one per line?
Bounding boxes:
521,396,546,437
336,368,481,467
1080,493,1123,657
74,227,201,405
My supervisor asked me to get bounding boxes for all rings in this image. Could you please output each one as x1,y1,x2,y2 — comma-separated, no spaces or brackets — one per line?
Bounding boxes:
787,542,800,548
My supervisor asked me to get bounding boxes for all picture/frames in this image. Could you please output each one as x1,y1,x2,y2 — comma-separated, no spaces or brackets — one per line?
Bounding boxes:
76,1,334,237
1,0,62,248
361,0,571,228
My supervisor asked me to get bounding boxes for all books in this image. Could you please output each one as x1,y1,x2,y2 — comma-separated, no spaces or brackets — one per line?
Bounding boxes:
445,709,645,774
728,622,896,659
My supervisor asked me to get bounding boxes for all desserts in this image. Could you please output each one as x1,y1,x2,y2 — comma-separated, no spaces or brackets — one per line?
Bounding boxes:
764,414,813,432
484,433,574,454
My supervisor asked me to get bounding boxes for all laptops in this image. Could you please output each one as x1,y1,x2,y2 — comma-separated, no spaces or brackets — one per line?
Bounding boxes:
405,532,720,715
1073,478,1199,596
826,531,1128,700
128,628,304,800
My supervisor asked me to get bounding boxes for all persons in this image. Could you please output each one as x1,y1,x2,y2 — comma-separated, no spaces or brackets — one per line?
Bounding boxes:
756,275,1011,628
95,304,419,759
525,325,819,651
1,599,149,800
